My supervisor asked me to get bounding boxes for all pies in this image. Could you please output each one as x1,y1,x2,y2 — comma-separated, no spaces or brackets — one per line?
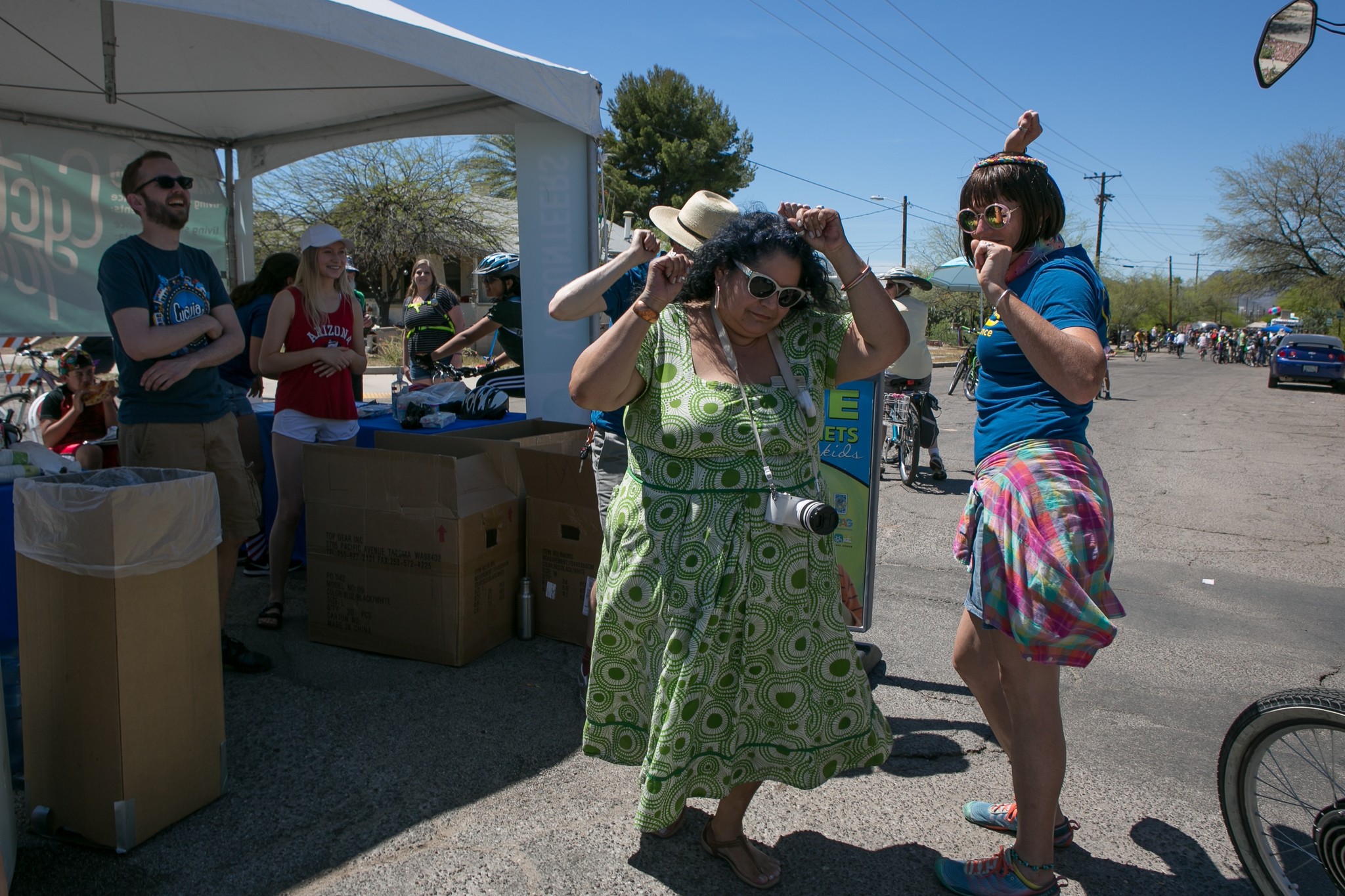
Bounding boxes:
81,381,115,406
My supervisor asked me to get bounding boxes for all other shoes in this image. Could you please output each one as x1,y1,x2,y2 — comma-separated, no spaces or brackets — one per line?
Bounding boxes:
221,634,273,674
880,460,886,479
1105,391,1111,400
929,453,947,480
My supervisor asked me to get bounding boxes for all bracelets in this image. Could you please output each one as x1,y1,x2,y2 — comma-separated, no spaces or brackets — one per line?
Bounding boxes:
840,265,870,291
633,300,659,325
991,288,1011,310
454,352,462,356
843,268,873,292
492,358,500,369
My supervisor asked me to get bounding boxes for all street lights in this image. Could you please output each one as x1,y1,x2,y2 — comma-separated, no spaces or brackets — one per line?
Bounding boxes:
870,194,909,269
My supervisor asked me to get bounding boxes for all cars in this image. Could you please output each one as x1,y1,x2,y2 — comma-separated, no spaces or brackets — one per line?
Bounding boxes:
1267,334,1345,394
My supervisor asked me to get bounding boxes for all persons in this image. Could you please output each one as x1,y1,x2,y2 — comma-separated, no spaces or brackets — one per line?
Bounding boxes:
219,251,302,574
97,149,275,674
39,348,117,470
345,255,374,402
570,203,910,888
414,252,527,412
548,190,1298,706
255,223,367,630
936,109,1126,896
402,259,464,386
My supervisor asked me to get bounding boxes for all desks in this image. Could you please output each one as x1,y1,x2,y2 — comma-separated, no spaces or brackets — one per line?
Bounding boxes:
0,441,73,779
253,407,526,564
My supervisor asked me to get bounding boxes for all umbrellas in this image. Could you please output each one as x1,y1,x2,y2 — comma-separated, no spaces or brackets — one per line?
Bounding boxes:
925,255,985,329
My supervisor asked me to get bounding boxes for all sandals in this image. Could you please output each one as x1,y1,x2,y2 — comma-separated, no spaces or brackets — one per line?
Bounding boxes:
700,814,780,888
652,806,689,838
255,602,285,630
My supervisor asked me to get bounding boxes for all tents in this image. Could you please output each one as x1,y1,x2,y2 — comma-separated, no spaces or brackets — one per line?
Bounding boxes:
1,1,602,427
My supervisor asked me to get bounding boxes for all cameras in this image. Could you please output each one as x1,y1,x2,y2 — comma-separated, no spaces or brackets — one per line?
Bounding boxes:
766,491,838,536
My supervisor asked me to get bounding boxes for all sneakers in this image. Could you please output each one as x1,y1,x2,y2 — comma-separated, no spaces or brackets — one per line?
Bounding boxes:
936,846,1068,896
242,550,301,575
963,800,1081,847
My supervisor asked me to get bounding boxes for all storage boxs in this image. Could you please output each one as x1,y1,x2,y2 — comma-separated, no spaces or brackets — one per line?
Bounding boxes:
13,466,228,855
302,415,602,669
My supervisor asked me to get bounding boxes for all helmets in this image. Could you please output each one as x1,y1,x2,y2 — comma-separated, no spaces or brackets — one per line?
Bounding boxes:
884,266,917,288
461,385,510,420
471,252,519,276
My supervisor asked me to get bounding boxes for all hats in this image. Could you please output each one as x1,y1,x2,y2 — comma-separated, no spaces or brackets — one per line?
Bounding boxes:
57,349,93,375
648,191,741,253
300,224,355,253
345,255,360,278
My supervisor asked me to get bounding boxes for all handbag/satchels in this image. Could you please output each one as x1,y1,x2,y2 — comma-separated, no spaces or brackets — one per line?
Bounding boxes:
919,392,941,450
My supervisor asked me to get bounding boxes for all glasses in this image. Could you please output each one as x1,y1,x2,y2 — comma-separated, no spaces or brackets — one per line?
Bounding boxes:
482,276,500,283
133,176,194,194
734,260,806,308
347,269,356,274
956,203,1021,234
886,283,901,289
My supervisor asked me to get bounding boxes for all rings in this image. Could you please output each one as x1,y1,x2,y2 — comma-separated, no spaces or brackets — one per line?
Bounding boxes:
985,242,993,251
1018,125,1028,132
816,205,824,209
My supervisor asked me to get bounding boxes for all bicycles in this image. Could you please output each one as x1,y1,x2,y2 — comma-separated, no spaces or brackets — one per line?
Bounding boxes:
1134,342,1148,362
410,355,493,385
1,345,69,446
1197,342,1208,361
1167,340,1184,359
1210,346,1271,367
883,376,927,486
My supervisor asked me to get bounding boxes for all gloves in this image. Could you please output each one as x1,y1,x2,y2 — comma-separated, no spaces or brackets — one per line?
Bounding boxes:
478,363,495,375
415,352,435,364
